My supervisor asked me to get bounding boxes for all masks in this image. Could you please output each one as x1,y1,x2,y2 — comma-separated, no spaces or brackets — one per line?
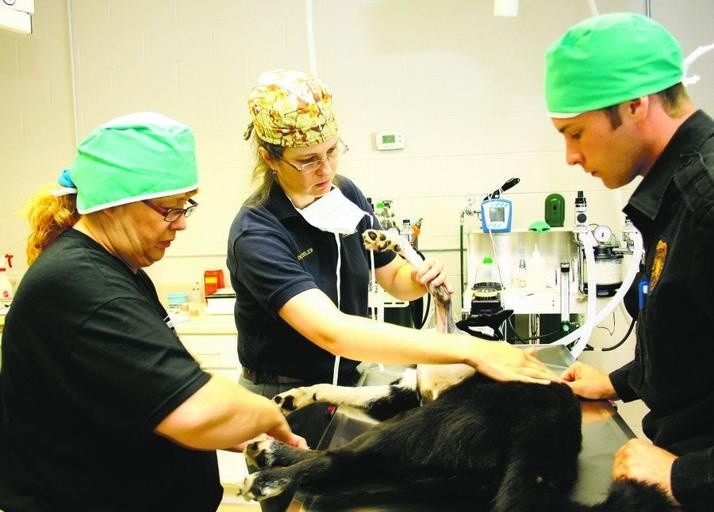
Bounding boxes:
284,181,379,261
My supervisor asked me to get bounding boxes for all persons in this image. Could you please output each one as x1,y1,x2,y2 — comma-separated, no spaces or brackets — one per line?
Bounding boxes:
228,70,565,473
0,113,308,511
559,11,714,511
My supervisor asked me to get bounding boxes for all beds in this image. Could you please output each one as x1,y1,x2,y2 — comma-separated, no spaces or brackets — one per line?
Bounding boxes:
282,342,680,511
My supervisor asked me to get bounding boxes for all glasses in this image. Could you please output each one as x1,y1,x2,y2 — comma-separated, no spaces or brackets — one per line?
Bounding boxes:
143,198,197,224
266,136,350,174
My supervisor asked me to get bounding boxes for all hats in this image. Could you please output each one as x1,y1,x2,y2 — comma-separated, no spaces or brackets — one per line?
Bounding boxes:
248,72,337,148
545,13,684,120
73,113,199,215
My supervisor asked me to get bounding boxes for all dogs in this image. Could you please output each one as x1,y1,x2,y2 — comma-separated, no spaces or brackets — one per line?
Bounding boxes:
236,227,686,512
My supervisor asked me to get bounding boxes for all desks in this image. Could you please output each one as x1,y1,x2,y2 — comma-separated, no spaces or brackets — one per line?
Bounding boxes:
155,283,242,372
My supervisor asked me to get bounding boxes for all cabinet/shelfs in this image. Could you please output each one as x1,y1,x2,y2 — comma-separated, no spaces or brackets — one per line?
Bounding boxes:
465,222,593,317
